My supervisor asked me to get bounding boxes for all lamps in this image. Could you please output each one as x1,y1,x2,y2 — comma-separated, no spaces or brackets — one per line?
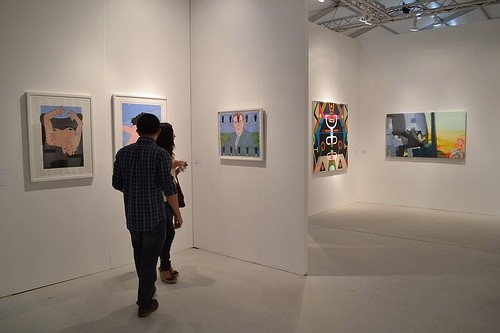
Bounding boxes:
359,14,378,28
432,11,441,26
410,14,419,31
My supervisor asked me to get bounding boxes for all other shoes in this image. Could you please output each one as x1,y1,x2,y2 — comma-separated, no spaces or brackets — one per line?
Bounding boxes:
158,265,179,284
137,298,159,318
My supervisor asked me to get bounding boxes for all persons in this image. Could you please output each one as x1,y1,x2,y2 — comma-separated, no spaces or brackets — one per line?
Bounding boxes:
112,112,183,318
154,123,187,284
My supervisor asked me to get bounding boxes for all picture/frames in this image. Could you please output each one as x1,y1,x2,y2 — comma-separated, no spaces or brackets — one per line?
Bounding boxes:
113,95,167,160
23,91,94,183
216,108,264,162
